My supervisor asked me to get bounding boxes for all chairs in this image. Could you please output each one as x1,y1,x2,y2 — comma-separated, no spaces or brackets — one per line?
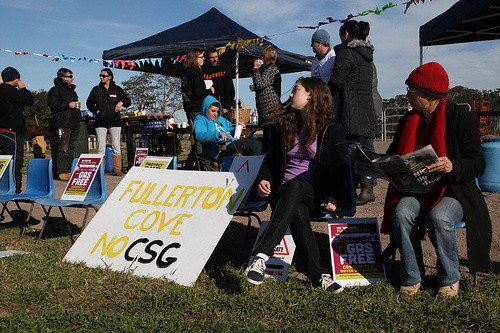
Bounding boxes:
0,158,55,240
310,156,357,222
390,177,479,288
222,156,267,257
35,158,107,245
193,128,242,170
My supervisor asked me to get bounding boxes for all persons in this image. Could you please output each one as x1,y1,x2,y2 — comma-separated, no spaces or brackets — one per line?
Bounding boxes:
193,95,238,161
201,47,235,120
46,67,83,182
380,62,493,297
310,20,383,205
251,46,281,128
181,49,215,126
86,68,131,176
0,67,34,194
244,77,354,295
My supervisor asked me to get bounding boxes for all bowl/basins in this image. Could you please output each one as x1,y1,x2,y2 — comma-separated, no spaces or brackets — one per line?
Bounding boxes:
173,124,181,129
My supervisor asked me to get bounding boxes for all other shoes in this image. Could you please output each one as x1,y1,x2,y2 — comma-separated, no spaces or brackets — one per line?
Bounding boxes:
399,283,420,296
59,173,71,180
436,280,459,302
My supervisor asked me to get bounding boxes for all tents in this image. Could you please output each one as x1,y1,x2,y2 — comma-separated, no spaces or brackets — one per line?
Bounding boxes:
419,0,500,67
103,7,315,139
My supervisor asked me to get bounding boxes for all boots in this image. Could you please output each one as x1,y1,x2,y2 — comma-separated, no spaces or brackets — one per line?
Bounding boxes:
354,172,375,206
113,153,124,176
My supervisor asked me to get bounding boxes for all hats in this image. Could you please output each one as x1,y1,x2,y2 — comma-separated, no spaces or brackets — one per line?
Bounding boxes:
312,29,330,45
2,67,20,83
404,61,449,97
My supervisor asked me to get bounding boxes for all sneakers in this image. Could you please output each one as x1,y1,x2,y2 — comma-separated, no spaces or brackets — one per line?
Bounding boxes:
246,257,266,285
306,273,344,294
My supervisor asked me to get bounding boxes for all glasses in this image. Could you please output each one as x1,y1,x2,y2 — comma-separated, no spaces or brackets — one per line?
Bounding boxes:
405,86,424,96
198,56,205,60
64,75,73,79
99,74,110,78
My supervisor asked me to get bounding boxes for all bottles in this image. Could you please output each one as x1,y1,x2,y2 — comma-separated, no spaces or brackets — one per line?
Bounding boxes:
252,112,256,124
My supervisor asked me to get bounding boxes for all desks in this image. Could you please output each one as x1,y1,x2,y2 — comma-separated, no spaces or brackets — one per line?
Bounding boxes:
161,128,191,161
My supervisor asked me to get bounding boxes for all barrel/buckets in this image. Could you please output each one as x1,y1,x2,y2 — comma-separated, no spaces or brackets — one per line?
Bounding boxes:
478,138,500,191
105,145,114,173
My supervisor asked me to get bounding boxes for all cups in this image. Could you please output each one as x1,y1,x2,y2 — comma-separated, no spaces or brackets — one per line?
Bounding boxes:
182,122,186,128
251,83,255,91
234,125,243,139
74,102,80,108
